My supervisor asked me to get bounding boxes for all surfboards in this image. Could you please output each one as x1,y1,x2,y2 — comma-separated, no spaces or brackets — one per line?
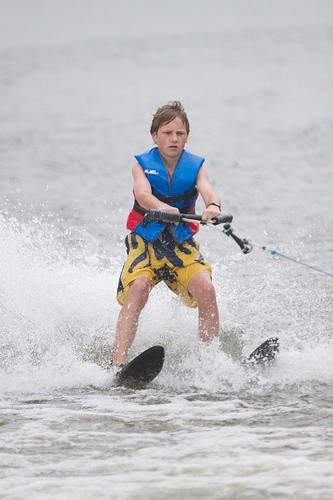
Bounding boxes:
117,345,166,383
247,338,279,366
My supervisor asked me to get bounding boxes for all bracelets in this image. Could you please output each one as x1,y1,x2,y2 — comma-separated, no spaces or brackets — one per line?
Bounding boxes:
205,201,221,212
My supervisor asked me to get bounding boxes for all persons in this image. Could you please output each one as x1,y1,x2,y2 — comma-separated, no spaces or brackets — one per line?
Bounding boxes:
106,98,223,372
